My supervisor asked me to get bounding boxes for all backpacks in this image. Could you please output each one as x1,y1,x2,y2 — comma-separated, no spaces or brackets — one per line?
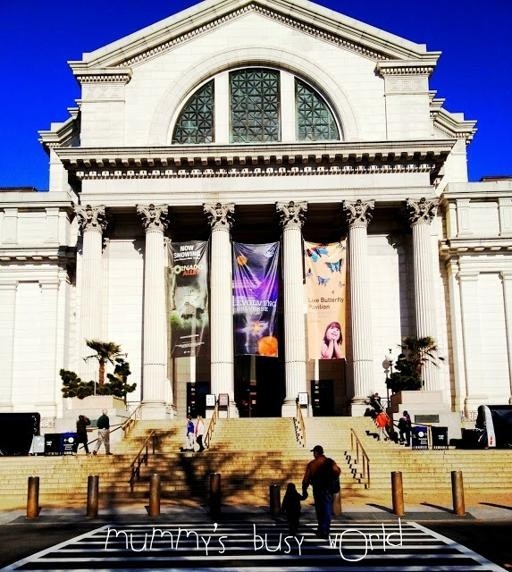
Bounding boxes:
320,461,335,485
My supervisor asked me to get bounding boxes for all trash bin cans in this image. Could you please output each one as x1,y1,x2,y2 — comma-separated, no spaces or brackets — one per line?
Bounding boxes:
411,425,448,450
44,433,79,456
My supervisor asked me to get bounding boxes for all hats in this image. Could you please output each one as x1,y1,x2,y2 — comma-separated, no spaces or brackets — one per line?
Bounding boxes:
310,444,324,452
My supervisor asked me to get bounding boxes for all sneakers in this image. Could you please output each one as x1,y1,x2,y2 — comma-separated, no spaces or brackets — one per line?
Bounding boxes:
93,450,114,455
317,529,331,539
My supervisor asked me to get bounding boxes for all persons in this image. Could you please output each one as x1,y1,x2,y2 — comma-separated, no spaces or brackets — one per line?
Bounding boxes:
397,410,413,447
71,415,93,454
194,415,205,452
320,322,343,359
91,408,113,455
281,483,307,541
185,415,195,451
302,445,341,539
375,410,391,441
